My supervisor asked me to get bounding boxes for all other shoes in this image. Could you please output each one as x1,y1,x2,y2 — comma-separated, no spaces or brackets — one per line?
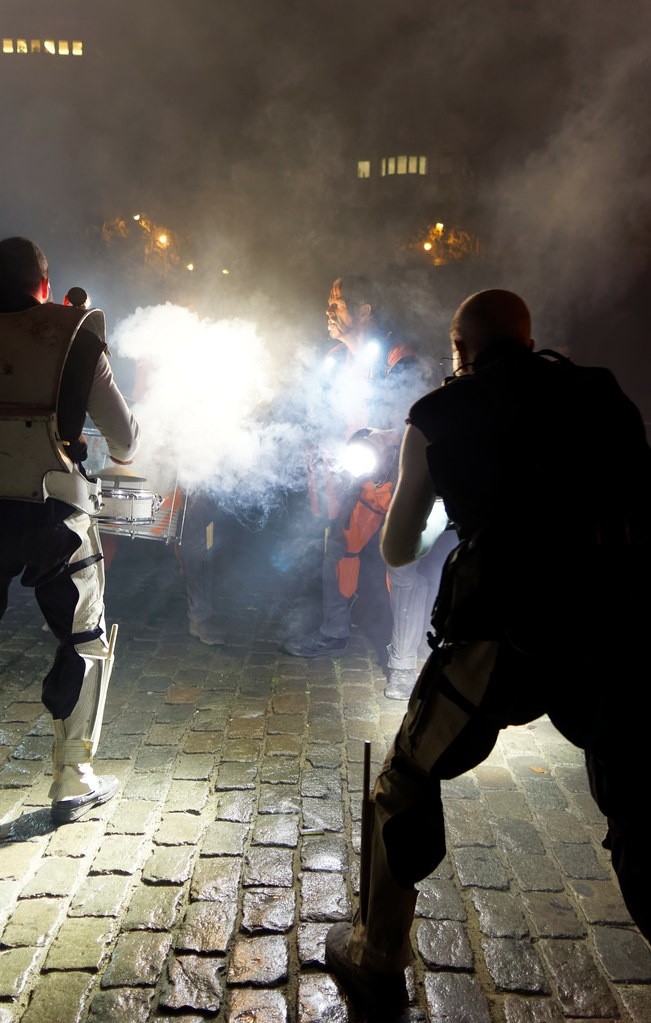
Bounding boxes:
325,921,409,1013
53,774,121,821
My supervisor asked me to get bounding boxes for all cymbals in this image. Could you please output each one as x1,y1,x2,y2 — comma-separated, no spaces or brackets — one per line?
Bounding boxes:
89,466,148,482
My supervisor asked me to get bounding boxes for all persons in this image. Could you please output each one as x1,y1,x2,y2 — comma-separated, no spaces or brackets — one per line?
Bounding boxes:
39,499,225,647
324,285,650,1011
2,237,140,822
279,274,455,703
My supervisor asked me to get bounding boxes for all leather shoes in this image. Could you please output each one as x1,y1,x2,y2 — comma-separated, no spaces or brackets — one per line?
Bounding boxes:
284,631,348,659
384,668,417,700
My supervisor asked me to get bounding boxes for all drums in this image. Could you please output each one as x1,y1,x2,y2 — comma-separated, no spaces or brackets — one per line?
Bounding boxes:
77,427,192,546
